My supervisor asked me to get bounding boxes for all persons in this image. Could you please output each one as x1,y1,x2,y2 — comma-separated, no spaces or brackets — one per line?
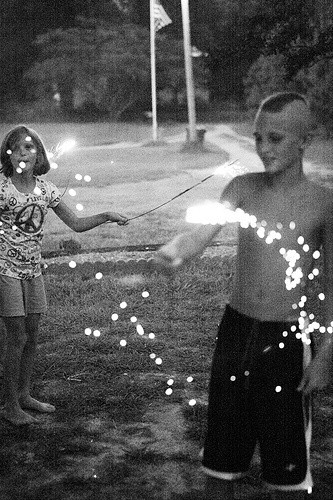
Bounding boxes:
146,90,332,500
0,124,129,427
